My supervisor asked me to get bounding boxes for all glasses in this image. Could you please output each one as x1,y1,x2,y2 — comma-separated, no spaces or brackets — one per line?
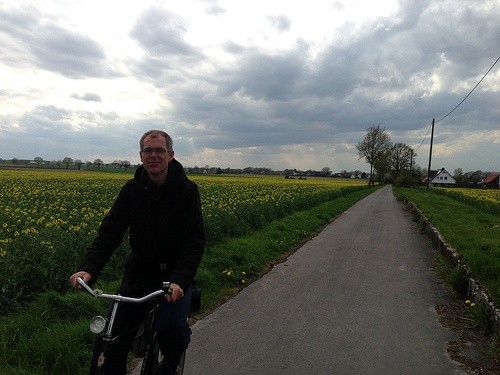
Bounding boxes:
141,149,170,155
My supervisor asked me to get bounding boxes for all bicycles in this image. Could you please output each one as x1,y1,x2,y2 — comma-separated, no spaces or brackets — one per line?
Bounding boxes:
77,277,186,375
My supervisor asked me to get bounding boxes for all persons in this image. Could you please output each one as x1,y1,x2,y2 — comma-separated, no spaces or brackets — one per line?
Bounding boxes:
70,130,205,375
